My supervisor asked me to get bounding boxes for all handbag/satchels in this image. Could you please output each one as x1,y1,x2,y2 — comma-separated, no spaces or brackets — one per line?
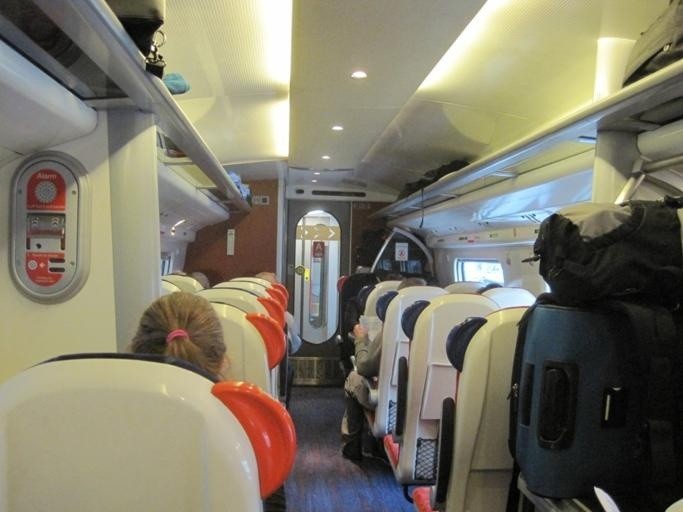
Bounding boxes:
106,0,166,80
622,0,683,86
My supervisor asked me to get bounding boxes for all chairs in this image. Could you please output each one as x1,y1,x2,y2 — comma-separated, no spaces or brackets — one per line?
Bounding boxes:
382,292,502,487
160,272,288,417
444,281,485,297
347,280,405,372
0,353,298,512
363,285,448,442
412,306,536,510
481,286,537,311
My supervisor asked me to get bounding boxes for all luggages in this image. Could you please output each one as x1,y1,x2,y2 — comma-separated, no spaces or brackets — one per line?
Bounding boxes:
507,301,681,496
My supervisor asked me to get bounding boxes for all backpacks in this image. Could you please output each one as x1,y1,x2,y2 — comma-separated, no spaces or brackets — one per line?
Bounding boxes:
533,195,683,305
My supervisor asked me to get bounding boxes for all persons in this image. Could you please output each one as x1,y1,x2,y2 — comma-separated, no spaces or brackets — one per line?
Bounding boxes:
256,272,303,410
477,283,502,294
340,277,425,462
190,271,211,289
128,291,286,512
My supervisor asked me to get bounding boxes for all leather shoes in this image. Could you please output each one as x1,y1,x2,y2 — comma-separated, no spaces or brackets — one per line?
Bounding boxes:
343,443,362,460
